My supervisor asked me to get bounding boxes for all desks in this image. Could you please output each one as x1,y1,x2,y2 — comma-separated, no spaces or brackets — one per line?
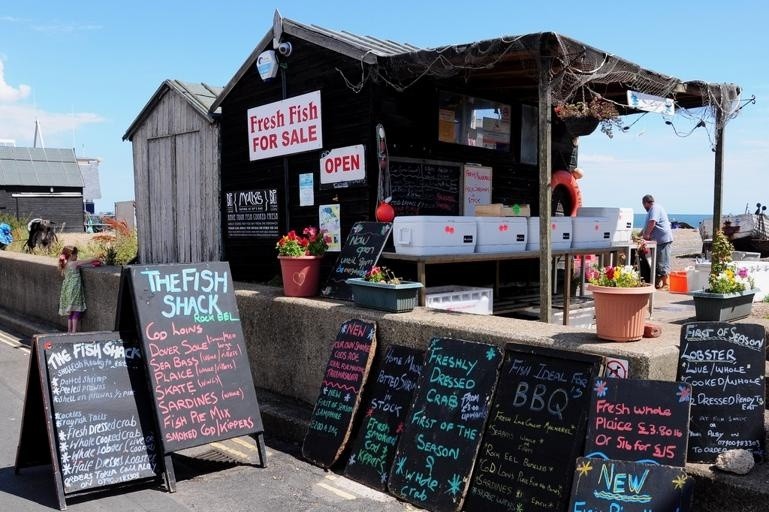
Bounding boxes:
381,238,661,324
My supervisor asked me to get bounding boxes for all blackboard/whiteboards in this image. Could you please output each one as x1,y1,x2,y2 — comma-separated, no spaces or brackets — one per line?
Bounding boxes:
460,342,607,512
568,457,696,512
345,345,425,492
16,330,176,498
675,321,766,464
302,317,378,467
585,377,692,467
320,221,393,302
387,337,505,512
115,262,265,455
389,157,463,217
463,166,493,216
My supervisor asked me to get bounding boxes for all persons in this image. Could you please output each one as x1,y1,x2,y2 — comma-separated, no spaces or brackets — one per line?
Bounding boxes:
0,223,14,252
636,194,675,292
551,103,602,216
58,244,104,333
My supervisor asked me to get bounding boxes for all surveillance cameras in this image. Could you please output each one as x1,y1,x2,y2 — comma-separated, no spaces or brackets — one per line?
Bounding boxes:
278,42,290,55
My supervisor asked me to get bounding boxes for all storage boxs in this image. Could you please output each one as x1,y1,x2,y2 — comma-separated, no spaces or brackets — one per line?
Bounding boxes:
528,216,572,251
476,216,528,254
572,217,612,249
420,284,494,314
576,206,634,244
393,215,476,257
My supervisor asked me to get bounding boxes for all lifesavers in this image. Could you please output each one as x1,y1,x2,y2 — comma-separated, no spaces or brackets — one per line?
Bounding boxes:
552,171,582,217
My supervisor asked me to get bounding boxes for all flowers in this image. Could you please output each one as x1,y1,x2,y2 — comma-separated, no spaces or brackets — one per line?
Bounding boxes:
273,226,330,256
708,228,754,294
364,266,401,284
554,98,626,139
590,262,647,287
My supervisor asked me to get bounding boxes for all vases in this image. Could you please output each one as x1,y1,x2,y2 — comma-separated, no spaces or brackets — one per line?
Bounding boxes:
278,255,321,298
588,287,655,343
345,276,423,314
558,117,600,137
688,289,755,326
